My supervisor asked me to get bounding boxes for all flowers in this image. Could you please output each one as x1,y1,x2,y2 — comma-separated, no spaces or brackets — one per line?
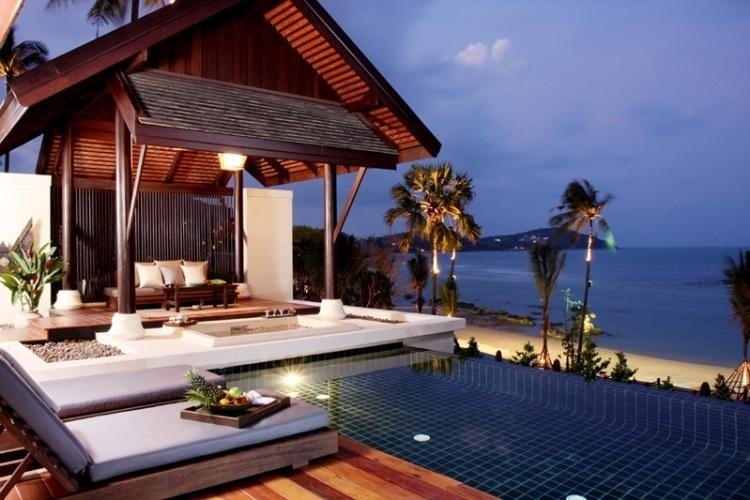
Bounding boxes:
1,238,69,311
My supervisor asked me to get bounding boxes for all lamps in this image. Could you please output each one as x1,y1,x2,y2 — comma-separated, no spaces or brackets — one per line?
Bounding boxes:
217,149,249,173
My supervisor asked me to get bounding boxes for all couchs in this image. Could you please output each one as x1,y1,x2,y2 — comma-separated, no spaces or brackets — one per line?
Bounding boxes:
101,262,240,311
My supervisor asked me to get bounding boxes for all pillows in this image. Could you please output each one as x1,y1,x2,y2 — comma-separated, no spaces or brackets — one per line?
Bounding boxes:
134,258,210,289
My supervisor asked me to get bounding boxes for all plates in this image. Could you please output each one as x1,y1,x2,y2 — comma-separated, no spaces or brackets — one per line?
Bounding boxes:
164,320,198,327
200,392,254,414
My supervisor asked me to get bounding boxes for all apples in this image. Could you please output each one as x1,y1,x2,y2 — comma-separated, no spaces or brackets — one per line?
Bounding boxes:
228,386,248,405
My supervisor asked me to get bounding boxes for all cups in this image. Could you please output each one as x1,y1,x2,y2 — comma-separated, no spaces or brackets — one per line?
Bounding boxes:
169,314,189,324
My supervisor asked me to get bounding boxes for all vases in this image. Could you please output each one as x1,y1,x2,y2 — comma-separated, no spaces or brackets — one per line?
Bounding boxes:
14,311,44,330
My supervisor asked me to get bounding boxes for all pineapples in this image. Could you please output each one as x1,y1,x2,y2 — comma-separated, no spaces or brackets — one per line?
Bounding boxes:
182,370,227,403
